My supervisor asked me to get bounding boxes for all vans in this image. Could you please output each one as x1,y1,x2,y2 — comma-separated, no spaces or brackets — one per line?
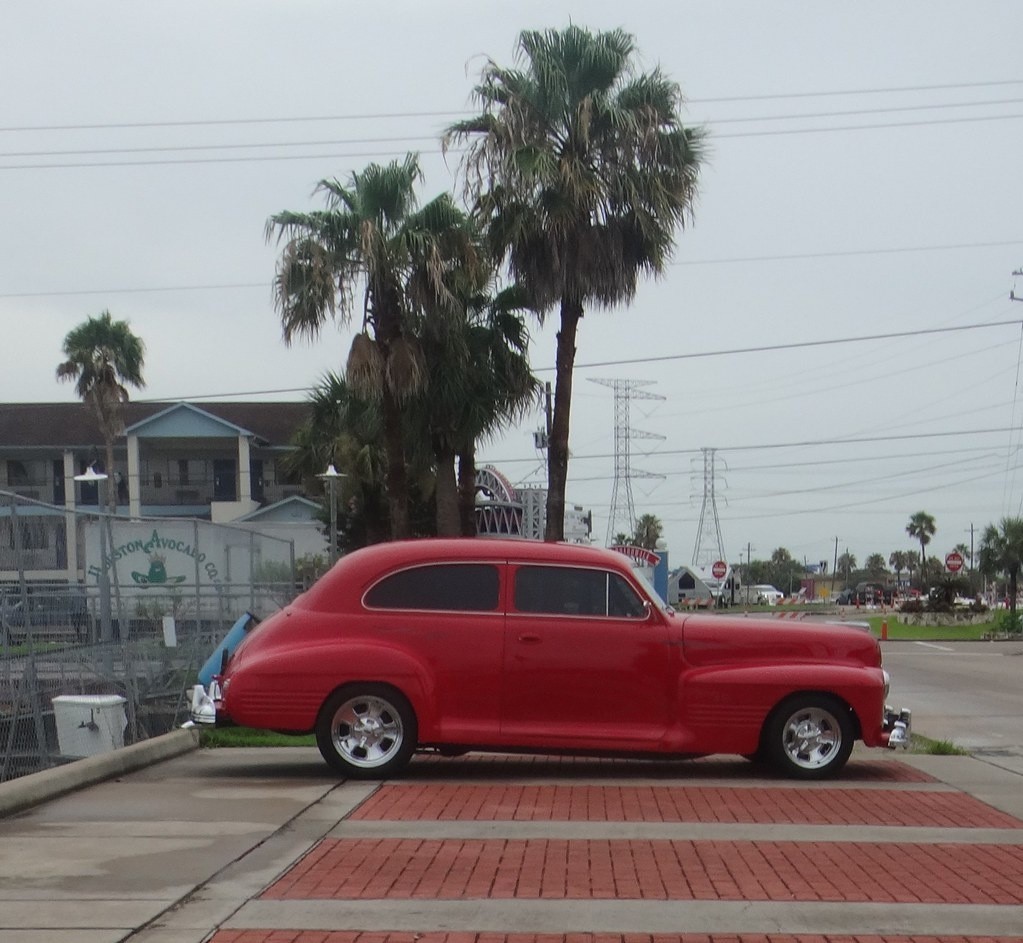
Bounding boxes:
858,582,883,604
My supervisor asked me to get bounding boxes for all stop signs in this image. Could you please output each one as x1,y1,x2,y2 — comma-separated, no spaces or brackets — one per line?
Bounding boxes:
946,554,962,571
712,561,727,579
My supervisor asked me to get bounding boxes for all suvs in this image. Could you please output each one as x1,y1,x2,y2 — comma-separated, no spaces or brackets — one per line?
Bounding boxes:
742,584,784,605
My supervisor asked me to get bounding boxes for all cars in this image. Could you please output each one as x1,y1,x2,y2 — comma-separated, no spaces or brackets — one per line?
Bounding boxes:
835,589,866,606
179,534,912,781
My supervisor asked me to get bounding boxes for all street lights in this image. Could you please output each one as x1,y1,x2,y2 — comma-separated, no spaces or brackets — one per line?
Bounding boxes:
74,460,114,695
313,463,348,569
